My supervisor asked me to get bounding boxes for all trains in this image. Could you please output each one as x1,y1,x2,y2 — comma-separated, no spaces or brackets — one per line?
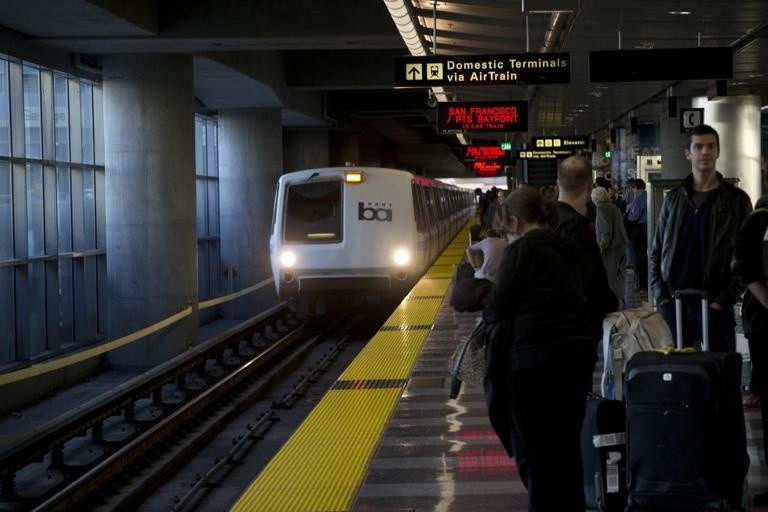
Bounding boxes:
269,163,479,303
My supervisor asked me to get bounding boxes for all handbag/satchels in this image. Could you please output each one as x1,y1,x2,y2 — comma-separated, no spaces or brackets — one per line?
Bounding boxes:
599,300,675,404
441,319,489,400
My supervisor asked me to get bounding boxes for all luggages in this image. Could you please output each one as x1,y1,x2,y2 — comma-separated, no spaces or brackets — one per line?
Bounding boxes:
581,393,631,512
620,286,744,512
